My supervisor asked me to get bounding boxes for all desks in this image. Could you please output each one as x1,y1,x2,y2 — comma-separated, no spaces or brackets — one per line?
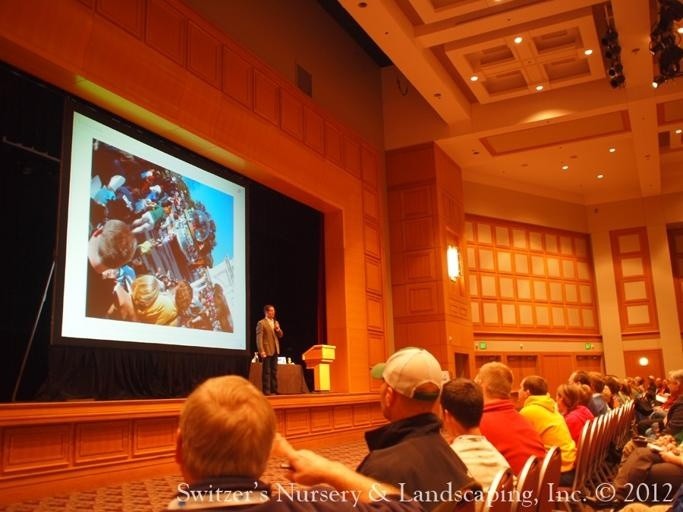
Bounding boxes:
248,362,310,394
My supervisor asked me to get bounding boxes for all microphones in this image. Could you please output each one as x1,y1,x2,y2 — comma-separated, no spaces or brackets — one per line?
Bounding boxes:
274,317,276,325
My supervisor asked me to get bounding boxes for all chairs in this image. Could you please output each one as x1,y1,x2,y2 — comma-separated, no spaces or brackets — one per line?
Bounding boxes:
426,391,648,512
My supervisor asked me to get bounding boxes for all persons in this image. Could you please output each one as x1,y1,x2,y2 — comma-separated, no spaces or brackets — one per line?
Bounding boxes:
257,305,283,396
163,345,683,512
86,168,231,336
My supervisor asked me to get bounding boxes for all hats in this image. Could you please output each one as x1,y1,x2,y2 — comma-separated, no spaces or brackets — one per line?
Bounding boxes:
371,345,444,400
